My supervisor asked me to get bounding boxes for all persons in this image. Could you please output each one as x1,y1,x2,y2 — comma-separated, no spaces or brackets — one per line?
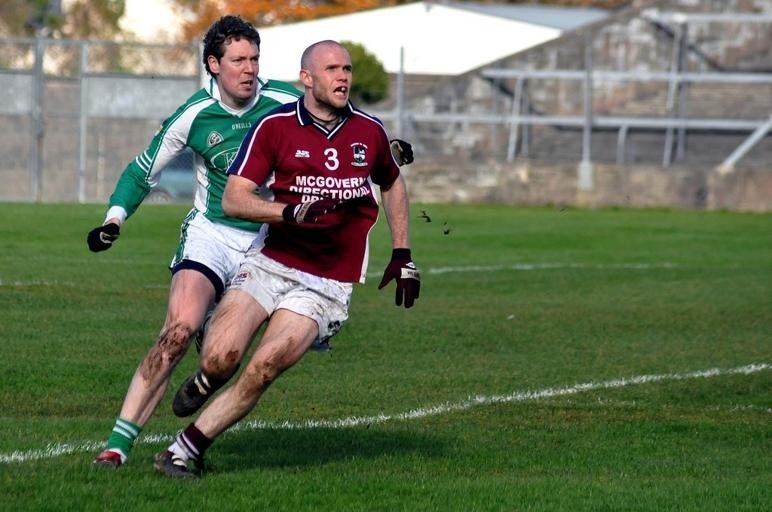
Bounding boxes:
87,13,415,471
147,37,423,483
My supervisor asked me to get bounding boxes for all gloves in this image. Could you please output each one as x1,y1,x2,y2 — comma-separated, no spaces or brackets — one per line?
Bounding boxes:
282,199,341,225
87,222,120,252
378,248,420,308
390,138,414,167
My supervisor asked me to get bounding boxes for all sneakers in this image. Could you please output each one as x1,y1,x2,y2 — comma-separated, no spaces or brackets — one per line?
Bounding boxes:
92,447,126,472
173,363,240,418
194,309,215,353
153,448,199,481
